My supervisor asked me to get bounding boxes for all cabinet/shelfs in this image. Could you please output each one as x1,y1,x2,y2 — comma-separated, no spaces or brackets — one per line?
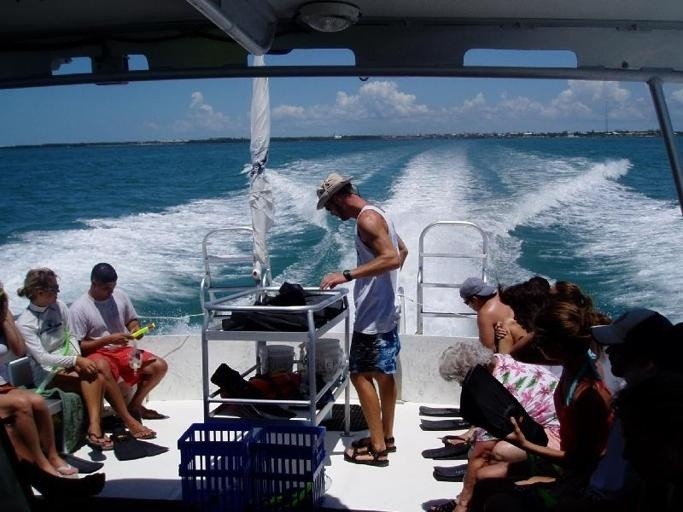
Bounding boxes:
198,276,355,445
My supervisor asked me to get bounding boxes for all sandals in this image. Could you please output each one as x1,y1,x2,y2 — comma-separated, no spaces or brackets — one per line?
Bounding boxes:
345,445,388,467
428,501,457,511
352,437,396,452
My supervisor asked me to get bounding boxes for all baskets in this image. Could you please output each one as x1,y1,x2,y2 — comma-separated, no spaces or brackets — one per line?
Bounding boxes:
178,423,253,503
251,425,325,508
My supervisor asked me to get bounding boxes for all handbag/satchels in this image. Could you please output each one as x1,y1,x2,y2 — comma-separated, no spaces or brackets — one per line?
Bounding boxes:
249,372,300,398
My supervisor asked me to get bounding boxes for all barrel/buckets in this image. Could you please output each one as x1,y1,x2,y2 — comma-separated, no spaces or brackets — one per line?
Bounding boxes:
255,342,298,383
296,336,350,393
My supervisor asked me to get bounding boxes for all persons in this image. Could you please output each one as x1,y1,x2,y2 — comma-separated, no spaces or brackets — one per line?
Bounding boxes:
0,282,78,479
13,267,158,449
69,262,168,439
441,277,682,512
315,173,408,467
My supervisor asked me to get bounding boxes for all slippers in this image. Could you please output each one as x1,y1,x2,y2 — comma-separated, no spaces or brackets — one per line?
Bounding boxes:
58,463,79,475
128,424,156,439
86,430,105,446
101,433,114,450
133,408,164,418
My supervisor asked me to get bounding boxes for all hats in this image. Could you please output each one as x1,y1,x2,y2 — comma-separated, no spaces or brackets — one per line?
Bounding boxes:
461,279,494,298
316,178,353,208
590,308,674,345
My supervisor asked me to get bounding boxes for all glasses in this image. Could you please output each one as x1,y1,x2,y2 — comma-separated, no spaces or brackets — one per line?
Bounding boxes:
464,297,473,306
324,204,333,210
32,284,58,292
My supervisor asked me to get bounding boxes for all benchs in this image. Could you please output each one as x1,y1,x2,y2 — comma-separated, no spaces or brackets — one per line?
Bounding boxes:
7,355,146,454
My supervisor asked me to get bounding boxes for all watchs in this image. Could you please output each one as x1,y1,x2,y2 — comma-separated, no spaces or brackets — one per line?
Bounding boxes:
342,270,350,280
